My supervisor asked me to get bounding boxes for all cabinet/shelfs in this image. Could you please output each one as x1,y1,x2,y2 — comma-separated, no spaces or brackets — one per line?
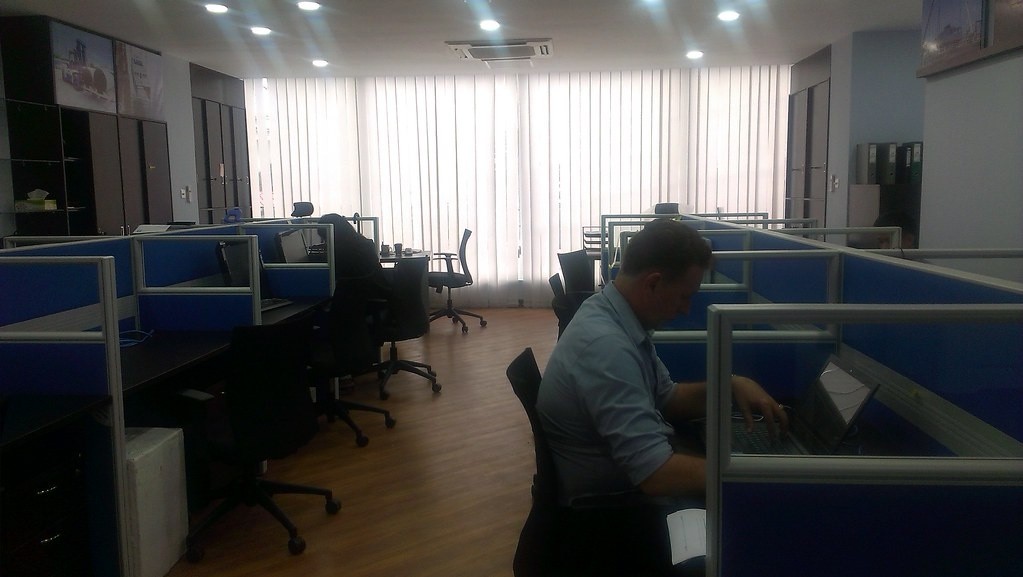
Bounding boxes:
126,426,190,577
783,77,831,244
0,16,175,235
0,444,90,577
850,182,921,249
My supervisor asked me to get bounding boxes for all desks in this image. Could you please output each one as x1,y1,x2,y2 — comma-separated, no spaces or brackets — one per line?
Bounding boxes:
601,211,1023,577
0,217,380,577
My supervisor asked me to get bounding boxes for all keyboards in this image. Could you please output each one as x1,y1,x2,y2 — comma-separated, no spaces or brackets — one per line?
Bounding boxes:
260,298,294,311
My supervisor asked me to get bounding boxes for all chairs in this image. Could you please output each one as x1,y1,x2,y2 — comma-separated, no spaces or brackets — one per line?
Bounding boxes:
371,258,442,401
504,348,680,577
173,309,342,563
549,248,597,344
305,267,398,449
429,229,487,333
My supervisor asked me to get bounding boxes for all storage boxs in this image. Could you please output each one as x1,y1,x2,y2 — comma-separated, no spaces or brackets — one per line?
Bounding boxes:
856,141,923,185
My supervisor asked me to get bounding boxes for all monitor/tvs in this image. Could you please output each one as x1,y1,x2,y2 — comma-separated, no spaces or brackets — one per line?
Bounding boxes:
217,240,268,287
275,229,310,264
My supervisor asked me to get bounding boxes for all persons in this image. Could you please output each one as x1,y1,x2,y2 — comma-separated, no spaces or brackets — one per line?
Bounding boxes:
535,218,790,576
319,214,388,400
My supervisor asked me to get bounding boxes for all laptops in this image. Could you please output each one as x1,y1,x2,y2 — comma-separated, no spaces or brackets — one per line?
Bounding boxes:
730,353,881,456
